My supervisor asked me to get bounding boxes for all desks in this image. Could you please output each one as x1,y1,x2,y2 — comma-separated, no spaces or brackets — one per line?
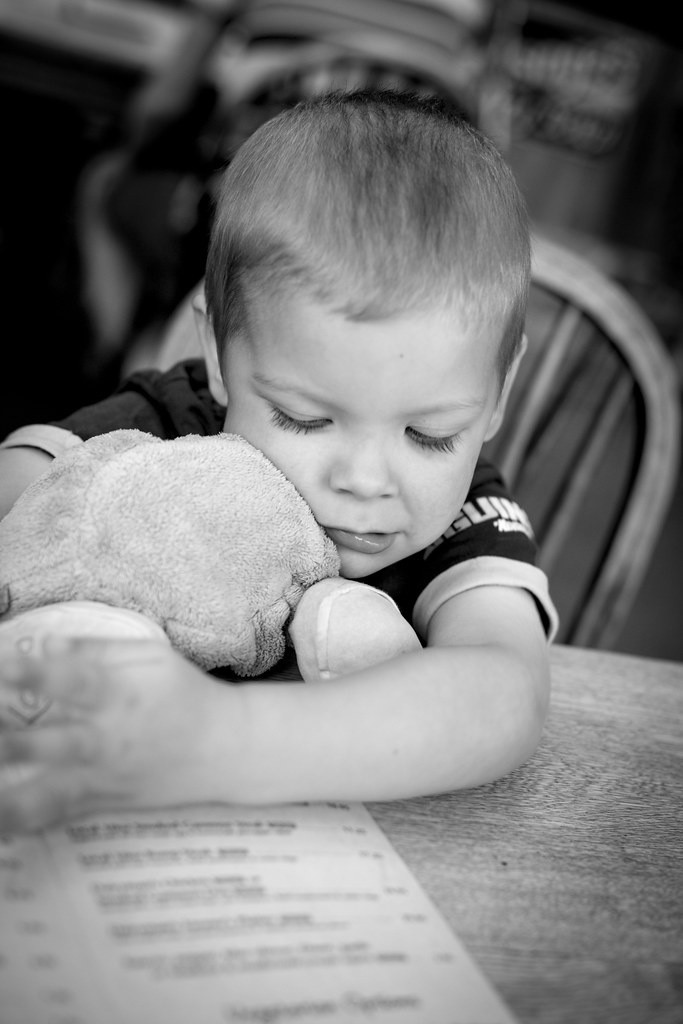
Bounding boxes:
1,639,681,1023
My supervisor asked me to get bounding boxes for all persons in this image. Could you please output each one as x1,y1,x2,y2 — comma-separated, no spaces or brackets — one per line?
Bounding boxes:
2,93,562,820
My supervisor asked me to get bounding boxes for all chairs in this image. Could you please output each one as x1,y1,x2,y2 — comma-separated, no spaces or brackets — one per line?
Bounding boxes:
154,227,681,651
112,4,483,407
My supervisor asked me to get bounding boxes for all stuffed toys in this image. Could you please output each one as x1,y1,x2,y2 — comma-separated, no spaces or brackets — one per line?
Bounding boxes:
1,429,423,684
